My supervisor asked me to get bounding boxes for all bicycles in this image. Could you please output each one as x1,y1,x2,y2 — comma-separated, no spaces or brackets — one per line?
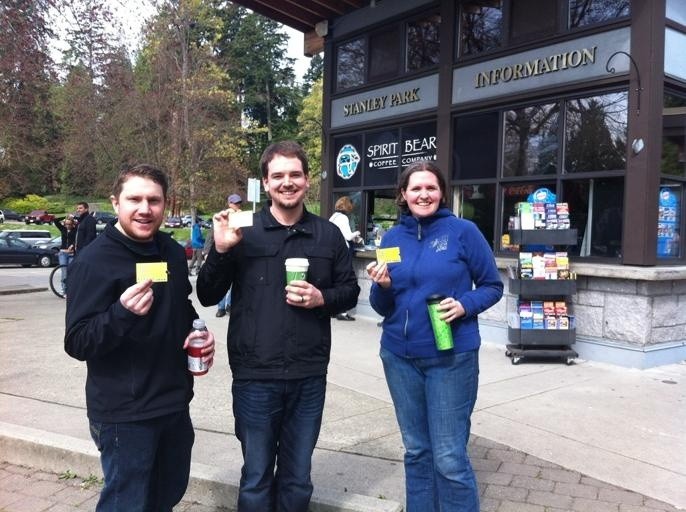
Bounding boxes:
50,264,71,299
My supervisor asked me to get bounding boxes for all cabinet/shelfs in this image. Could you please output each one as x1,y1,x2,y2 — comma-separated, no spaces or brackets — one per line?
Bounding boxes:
505,228,580,365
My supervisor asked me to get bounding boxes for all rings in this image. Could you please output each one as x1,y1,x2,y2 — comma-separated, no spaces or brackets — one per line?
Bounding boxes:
299,296,305,303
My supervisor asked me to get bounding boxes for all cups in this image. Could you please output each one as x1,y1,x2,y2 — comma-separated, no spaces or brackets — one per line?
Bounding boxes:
285,258,310,290
425,292,453,351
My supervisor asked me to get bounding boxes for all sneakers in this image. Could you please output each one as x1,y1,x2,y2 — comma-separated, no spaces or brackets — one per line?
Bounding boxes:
215,305,231,317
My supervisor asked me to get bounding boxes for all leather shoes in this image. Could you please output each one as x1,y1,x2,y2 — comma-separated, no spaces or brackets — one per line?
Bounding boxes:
332,314,355,321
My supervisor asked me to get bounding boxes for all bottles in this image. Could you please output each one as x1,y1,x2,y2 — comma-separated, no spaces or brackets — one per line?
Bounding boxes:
367,222,390,247
185,318,208,375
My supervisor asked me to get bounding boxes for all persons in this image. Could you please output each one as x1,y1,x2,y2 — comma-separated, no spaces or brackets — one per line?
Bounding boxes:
67,201,96,257
188,216,203,277
328,195,359,320
195,140,360,512
53,215,78,296
365,160,502,512
216,193,242,317
64,164,214,512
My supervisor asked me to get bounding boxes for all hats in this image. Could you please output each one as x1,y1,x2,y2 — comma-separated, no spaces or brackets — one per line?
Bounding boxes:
228,195,242,203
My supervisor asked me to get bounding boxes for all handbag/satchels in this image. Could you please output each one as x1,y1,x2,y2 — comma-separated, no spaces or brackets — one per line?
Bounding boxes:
352,236,365,248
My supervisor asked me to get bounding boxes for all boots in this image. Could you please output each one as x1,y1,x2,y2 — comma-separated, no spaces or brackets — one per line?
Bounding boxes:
188,267,201,275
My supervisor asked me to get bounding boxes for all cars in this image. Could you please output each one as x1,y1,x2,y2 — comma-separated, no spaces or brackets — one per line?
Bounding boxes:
0,204,212,267
